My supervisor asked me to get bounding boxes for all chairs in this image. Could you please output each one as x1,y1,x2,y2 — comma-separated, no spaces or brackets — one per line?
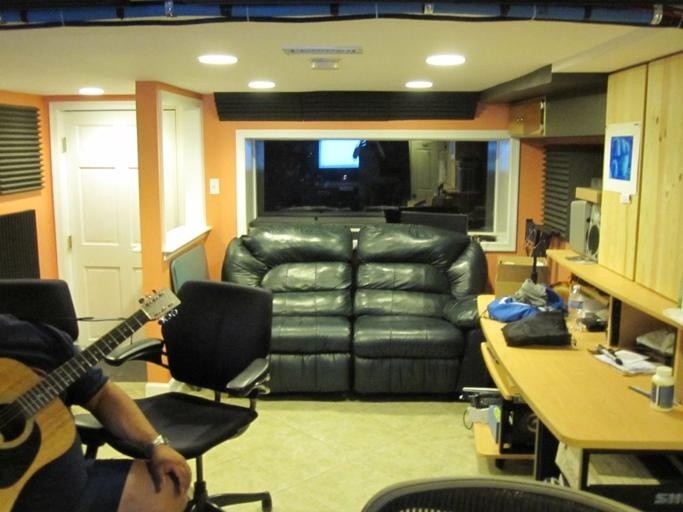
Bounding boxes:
0,279,105,459
101,279,274,512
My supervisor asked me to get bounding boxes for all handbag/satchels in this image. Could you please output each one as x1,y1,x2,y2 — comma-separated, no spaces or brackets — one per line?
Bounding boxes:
501,310,570,346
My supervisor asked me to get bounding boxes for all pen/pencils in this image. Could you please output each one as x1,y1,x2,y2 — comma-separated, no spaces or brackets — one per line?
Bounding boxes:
629,386,651,397
598,347,623,365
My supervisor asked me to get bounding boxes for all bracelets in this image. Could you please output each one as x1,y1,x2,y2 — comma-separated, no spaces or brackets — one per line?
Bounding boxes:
143,434,169,453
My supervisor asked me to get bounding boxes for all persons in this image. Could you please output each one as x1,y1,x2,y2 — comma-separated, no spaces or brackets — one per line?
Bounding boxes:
0,312,195,511
265,143,323,209
352,139,386,208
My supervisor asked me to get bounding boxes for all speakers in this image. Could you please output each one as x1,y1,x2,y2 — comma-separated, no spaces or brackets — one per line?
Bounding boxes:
569,200,592,256
586,203,601,262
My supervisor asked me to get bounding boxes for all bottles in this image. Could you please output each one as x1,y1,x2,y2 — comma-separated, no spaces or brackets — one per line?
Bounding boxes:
650,366,674,412
568,286,585,333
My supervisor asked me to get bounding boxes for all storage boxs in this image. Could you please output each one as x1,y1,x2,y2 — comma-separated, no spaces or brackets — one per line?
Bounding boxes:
494,256,548,297
554,441,661,512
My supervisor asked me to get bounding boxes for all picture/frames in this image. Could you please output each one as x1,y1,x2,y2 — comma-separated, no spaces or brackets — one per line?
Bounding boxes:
602,119,643,195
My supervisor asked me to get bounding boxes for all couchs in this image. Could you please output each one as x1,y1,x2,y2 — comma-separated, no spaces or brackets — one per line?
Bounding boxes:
221,222,488,396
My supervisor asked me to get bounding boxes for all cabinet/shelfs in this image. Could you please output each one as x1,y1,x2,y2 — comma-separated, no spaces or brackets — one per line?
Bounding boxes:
472,247,683,512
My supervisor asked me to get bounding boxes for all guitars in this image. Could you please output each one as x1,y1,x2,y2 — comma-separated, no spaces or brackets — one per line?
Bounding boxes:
0,288,181,512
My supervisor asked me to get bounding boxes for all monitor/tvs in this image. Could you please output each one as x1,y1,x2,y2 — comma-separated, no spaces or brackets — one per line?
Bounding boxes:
313,139,368,172
